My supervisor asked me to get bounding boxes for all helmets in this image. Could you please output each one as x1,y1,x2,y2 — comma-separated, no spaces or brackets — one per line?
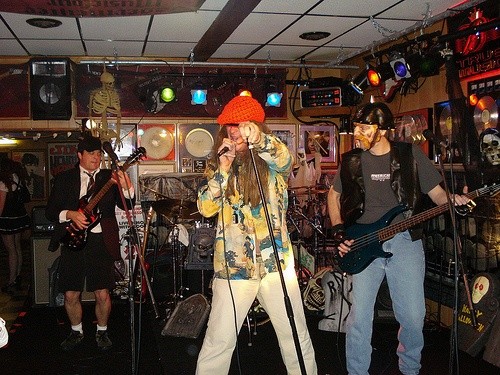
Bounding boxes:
350,102,397,129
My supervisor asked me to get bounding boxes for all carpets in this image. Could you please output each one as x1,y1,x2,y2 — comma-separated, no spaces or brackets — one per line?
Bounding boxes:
0,288,500,375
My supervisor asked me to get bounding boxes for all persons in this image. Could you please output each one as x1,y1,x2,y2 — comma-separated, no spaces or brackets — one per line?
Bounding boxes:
45,137,136,350
0,158,31,292
327,100,476,375
21,153,44,198
306,131,329,157
195,99,317,375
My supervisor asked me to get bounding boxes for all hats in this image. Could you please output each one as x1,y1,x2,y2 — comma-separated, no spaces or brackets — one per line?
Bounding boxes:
78,137,105,154
218,95,265,125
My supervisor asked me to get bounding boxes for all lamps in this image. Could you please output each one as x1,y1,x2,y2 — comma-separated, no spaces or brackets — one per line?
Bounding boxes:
188,76,208,106
350,60,376,95
367,54,395,88
405,37,444,76
136,72,178,113
263,77,283,107
390,43,424,82
233,76,253,98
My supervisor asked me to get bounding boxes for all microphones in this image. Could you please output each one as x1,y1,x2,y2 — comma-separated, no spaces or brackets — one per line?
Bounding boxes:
422,129,450,151
103,140,124,172
216,146,230,157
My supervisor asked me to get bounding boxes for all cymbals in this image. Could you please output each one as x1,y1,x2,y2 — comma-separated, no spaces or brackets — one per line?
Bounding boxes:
287,184,322,191
296,189,328,196
151,198,203,221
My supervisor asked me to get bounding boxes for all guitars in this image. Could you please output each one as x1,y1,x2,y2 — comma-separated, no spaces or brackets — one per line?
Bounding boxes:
335,178,500,276
53,146,147,252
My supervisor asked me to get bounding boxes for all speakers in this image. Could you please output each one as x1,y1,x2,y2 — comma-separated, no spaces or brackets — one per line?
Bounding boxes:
140,254,189,297
30,236,96,304
29,57,72,120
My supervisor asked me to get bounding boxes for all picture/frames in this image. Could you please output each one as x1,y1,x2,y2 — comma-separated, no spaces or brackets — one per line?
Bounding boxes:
265,123,298,165
11,148,48,202
97,122,136,161
298,124,336,163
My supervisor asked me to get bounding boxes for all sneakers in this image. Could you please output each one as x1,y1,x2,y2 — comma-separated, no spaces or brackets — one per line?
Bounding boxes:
60,330,85,350
96,329,112,350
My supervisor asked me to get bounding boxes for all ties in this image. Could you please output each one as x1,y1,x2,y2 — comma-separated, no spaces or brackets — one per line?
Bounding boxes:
84,171,97,221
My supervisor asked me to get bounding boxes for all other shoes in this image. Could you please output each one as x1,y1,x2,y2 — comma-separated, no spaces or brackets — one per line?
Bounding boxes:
3,276,22,289
